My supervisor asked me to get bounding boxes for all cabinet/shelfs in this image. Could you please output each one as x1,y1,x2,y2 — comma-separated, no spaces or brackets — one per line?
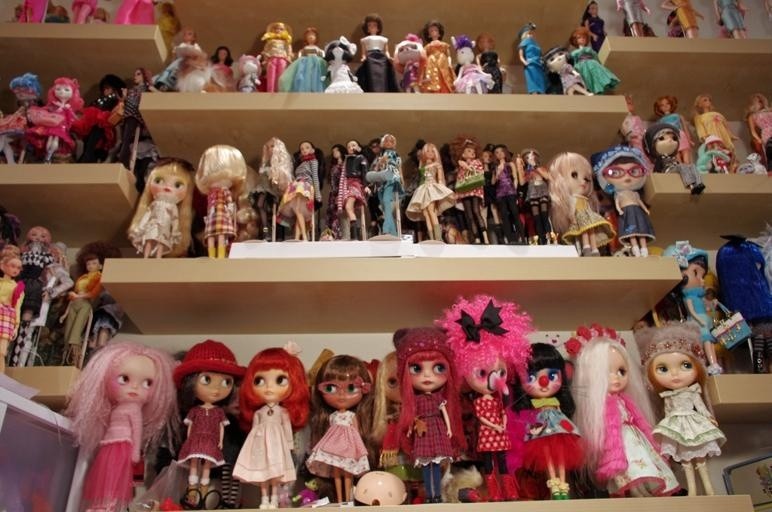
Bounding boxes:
0,0,772,512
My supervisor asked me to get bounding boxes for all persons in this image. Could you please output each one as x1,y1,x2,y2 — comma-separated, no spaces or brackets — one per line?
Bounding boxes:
1,0,771,512
0,0,772,512
